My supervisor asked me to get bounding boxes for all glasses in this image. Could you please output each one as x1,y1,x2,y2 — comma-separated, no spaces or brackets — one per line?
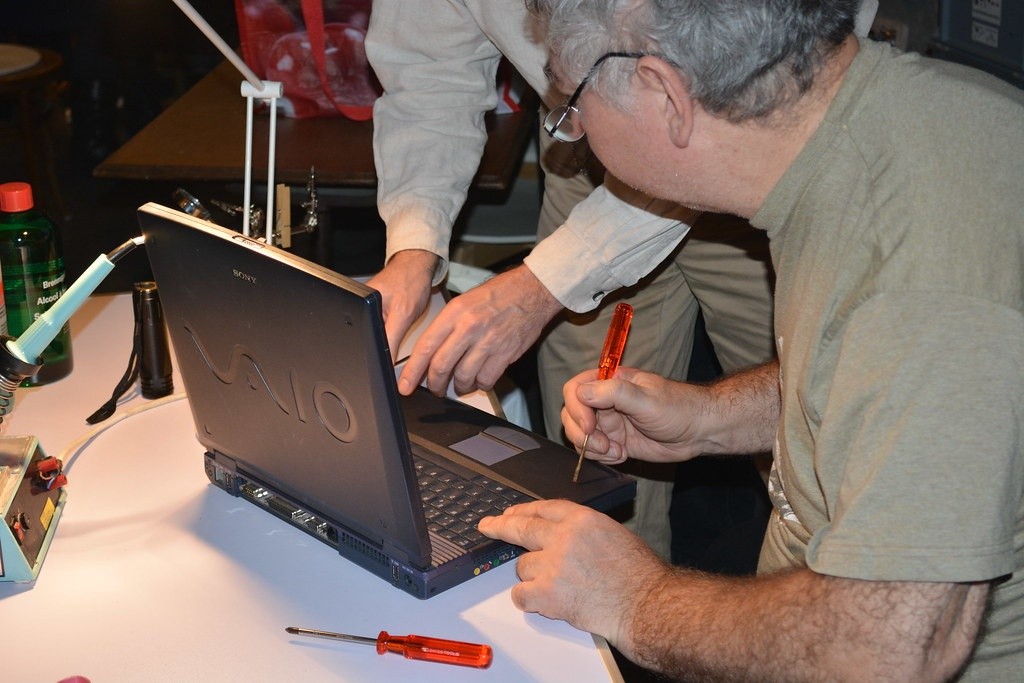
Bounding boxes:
538,46,686,145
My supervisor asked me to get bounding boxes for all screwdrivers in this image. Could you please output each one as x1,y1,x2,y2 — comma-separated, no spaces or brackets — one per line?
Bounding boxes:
571,302,635,485
285,626,494,670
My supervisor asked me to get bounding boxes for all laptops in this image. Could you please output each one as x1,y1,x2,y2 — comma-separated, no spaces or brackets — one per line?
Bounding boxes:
136,201,636,601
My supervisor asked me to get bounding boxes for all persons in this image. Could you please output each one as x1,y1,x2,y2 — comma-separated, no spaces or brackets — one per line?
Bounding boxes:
358,1,881,569
470,0,1024,683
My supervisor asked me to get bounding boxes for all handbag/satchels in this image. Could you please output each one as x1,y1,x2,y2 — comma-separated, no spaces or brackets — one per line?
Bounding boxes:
231,0,379,122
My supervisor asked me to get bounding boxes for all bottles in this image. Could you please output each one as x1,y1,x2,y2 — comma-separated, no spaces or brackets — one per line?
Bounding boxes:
1,181,74,387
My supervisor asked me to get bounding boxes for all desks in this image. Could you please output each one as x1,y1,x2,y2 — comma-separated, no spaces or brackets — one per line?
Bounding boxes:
93,46,537,267
0,43,60,86
0,268,627,683
